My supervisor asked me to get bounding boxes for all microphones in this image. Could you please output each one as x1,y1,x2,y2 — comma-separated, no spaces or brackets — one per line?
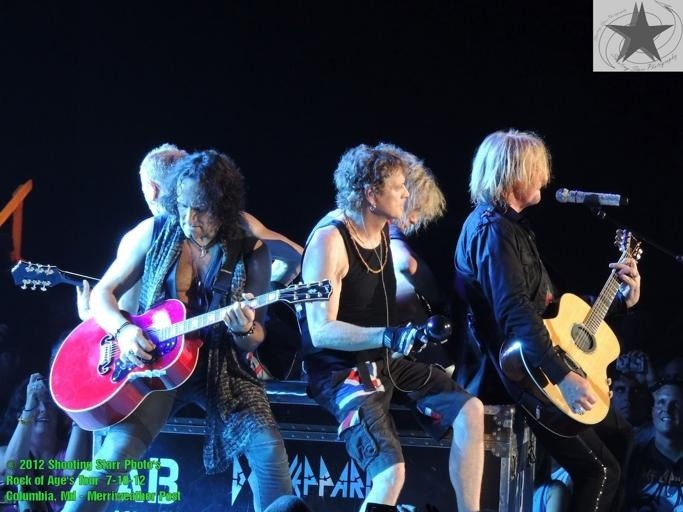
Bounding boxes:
553,187,632,210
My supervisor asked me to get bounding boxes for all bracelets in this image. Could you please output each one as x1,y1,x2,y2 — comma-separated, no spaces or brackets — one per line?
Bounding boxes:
225,321,257,338
113,320,132,344
17,414,35,425
71,421,77,427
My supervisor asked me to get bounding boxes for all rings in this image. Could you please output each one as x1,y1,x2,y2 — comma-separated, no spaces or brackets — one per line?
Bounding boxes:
33,385,37,390
575,407,585,415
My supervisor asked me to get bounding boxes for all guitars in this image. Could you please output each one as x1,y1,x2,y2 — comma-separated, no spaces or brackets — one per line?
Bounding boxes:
49,280,333,432
500,227,643,438
10,257,302,383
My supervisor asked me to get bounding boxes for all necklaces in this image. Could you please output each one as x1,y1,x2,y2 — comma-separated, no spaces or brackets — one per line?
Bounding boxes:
343,209,389,274
187,235,220,257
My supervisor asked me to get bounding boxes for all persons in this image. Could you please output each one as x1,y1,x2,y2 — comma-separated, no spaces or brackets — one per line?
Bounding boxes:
75,143,306,382
524,350,683,512
455,129,628,512
60,147,294,512
375,142,453,376
297,144,486,512
0,371,93,512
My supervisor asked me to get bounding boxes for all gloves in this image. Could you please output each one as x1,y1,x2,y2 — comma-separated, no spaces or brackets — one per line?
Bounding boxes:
383,325,446,364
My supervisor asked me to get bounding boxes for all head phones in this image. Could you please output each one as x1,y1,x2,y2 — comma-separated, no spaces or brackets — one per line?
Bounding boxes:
405,316,457,377
